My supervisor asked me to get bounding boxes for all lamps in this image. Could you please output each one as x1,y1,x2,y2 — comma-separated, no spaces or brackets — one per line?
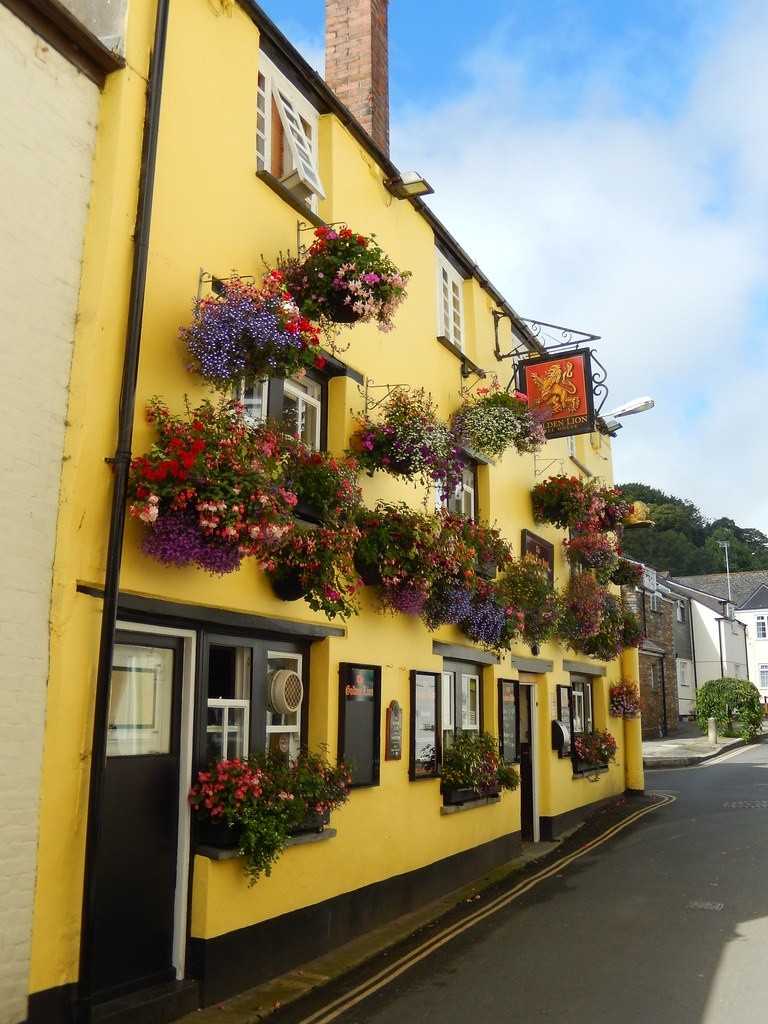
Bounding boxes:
595,395,655,419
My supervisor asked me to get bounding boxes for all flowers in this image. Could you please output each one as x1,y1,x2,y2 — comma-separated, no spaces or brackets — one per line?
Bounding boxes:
103,222,646,662
609,677,647,721
445,729,523,798
573,731,619,765
266,742,358,826
188,753,306,888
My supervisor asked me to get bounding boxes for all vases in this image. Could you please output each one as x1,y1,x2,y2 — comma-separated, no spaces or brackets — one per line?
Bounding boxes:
385,454,419,475
289,805,329,834
352,549,384,584
267,565,317,601
197,818,243,849
316,294,359,322
611,573,630,585
